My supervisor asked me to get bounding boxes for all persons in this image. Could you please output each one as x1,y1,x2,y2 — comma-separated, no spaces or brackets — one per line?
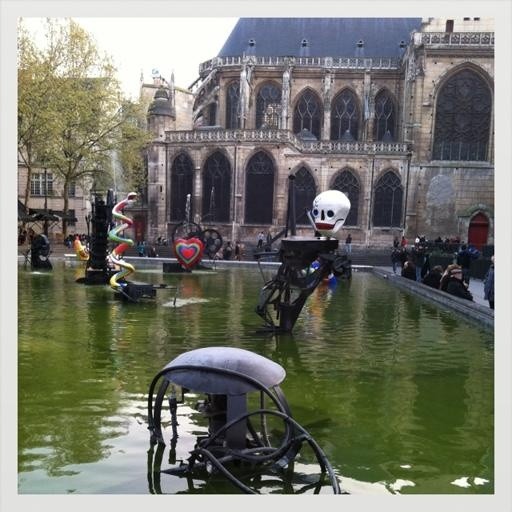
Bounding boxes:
394,236,400,251
398,247,411,268
345,233,352,256
390,247,399,272
401,256,417,281
423,264,443,289
265,245,272,262
440,263,469,292
209,241,241,260
137,235,168,257
456,244,473,290
414,235,460,246
64,233,91,248
447,268,473,301
461,240,466,250
18,223,37,244
401,236,407,251
482,253,494,309
421,254,431,278
257,231,264,247
